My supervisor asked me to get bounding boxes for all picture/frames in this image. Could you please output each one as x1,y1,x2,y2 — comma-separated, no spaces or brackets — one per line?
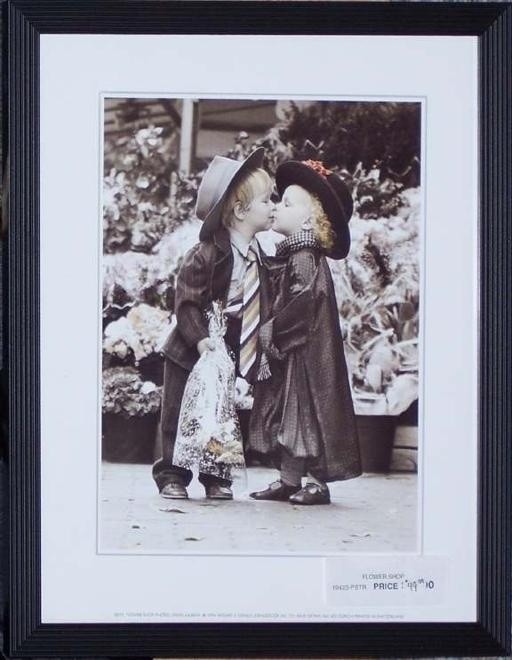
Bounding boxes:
1,0,512,658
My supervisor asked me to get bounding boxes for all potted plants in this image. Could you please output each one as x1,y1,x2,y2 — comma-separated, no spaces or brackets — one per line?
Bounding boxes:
99,250,419,472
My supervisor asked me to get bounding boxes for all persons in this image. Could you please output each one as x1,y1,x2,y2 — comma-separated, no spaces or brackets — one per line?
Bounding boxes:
243,157,367,507
147,141,276,502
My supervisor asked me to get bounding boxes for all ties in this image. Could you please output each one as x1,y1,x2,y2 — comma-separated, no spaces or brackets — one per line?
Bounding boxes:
238,251,262,376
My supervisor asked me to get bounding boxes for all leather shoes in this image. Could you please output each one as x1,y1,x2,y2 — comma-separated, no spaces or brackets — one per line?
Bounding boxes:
160,481,189,499
289,483,331,505
206,483,233,500
250,480,302,501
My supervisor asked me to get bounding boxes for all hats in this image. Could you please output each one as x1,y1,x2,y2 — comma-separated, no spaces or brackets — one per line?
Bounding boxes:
193,146,265,241
275,160,355,260
